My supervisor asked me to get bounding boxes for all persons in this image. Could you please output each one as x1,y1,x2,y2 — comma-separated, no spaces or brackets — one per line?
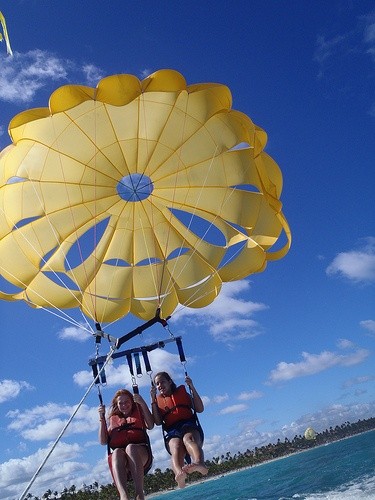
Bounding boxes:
151,372,208,489
97,388,155,500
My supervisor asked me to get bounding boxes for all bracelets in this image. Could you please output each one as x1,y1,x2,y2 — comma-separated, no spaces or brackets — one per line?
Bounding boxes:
100,417,104,422
